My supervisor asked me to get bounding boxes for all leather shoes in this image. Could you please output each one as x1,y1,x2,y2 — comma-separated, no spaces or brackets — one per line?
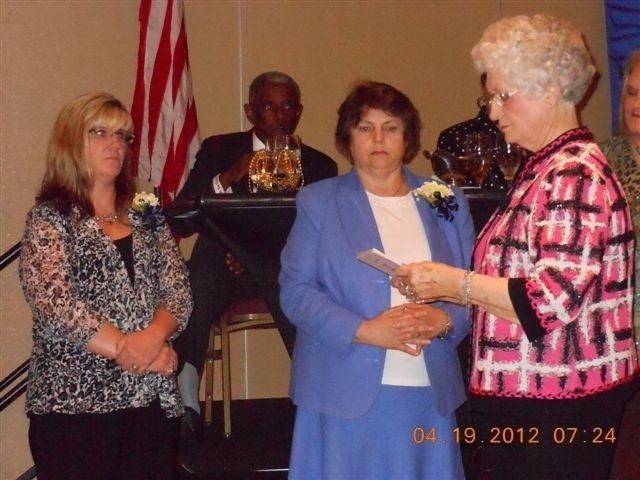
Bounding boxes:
178,406,203,437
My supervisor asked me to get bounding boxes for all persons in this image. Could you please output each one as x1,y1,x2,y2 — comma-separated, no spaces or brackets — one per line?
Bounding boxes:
159,70,338,444
279,77,483,479
592,46,639,346
396,13,639,479
19,91,193,478
433,65,532,186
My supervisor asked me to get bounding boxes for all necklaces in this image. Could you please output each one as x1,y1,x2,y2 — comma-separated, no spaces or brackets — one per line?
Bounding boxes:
91,212,120,226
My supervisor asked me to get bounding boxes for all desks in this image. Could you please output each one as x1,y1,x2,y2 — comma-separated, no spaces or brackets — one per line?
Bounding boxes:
193,185,507,361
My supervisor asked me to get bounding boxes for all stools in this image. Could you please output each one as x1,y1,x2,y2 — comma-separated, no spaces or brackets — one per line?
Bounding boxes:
204,295,279,443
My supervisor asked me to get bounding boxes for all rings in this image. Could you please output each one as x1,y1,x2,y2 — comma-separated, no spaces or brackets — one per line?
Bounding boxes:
404,286,412,296
131,364,139,371
168,367,173,375
412,295,422,304
400,280,404,286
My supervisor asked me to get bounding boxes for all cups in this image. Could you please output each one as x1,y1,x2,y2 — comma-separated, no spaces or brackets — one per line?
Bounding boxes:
264,136,300,176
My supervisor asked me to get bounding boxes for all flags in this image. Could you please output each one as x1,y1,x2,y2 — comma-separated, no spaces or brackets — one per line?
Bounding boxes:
125,0,206,251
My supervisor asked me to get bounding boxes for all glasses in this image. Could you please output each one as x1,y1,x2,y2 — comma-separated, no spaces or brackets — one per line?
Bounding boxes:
477,89,519,106
253,101,298,112
90,127,136,144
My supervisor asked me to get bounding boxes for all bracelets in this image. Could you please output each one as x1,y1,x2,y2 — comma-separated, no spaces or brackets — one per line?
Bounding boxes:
438,308,452,341
464,266,477,309
